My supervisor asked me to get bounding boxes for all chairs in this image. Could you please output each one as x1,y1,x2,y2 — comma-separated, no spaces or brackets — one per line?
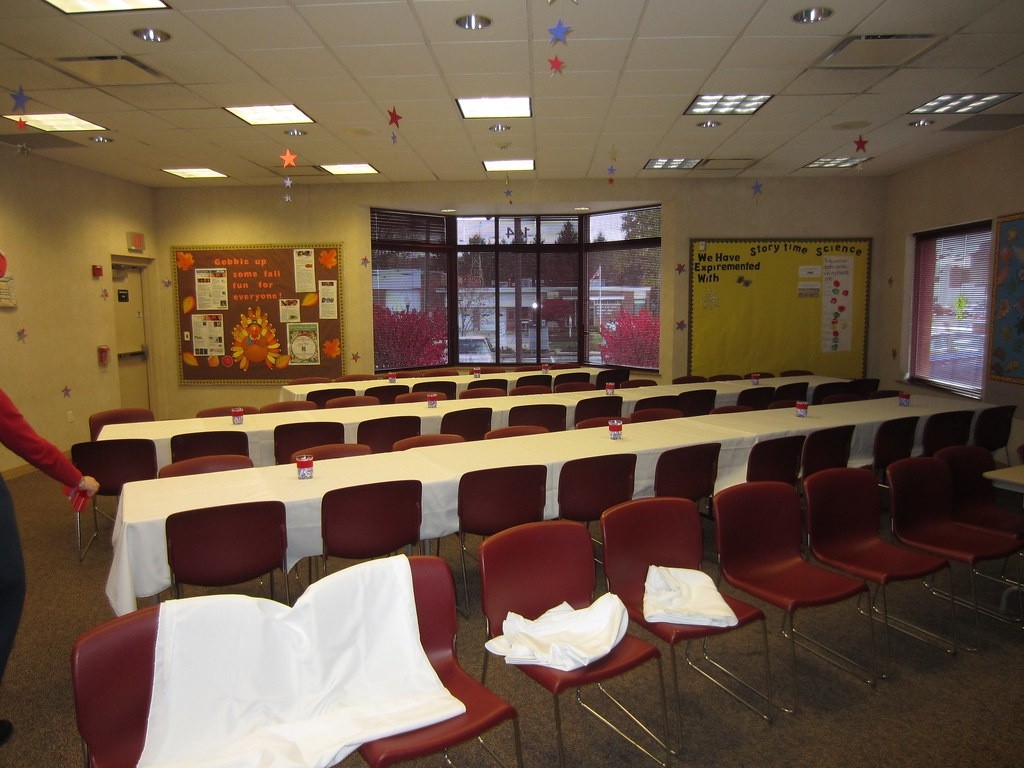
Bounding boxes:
70,361,1024,768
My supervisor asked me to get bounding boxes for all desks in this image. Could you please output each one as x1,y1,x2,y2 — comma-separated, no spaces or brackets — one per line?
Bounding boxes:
115,390,1003,603
90,374,855,441
281,366,616,396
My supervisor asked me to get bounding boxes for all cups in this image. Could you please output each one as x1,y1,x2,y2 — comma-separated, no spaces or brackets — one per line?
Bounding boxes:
605,382,615,396
542,364,549,374
795,401,808,417
473,367,481,378
427,394,438,408
387,372,397,383
296,455,314,479
607,419,623,440
898,391,911,406
751,373,761,385
231,407,244,424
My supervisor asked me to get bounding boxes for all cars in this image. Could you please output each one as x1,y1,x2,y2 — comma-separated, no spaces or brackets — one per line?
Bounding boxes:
929,332,985,386
932,302,953,316
962,304,986,320
458,336,495,362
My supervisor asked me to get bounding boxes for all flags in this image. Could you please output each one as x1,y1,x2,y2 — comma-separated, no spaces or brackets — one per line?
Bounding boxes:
590,267,601,290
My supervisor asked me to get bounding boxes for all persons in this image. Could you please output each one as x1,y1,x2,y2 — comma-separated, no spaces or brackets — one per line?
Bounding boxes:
0,252,100,748
